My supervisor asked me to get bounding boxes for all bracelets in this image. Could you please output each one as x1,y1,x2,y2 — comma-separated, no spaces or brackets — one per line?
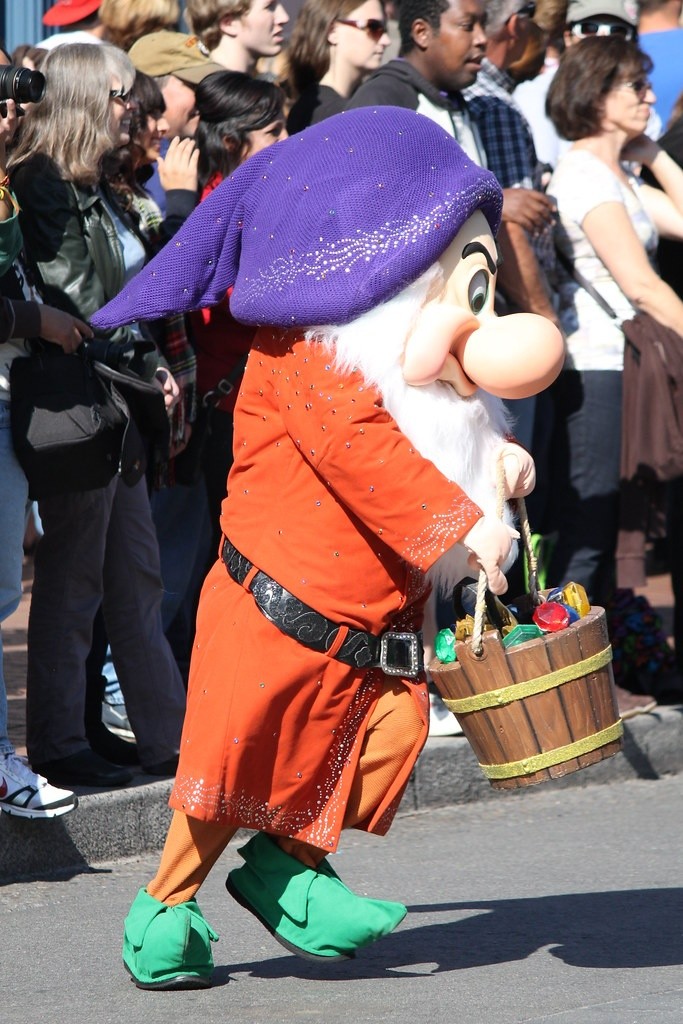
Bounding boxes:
0,172,10,200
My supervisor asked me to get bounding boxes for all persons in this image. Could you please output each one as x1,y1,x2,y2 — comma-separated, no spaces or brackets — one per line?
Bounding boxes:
85,105,567,991
0,0,683,819
545,35,683,605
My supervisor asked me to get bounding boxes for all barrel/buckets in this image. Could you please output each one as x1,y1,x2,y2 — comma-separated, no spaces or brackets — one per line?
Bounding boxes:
427,587,623,789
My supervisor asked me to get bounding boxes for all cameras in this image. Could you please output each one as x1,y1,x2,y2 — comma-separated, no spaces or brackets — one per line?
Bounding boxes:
0,64,46,103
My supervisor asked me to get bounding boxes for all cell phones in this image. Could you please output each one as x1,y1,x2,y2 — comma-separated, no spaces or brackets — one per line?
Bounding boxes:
0,99,25,118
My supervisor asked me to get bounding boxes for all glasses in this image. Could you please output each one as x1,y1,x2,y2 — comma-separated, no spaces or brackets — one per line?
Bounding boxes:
504,0,536,25
608,79,652,93
570,21,635,42
110,83,134,102
335,17,388,38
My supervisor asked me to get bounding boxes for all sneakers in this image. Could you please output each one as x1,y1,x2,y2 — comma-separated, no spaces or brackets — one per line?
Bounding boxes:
0,745,79,820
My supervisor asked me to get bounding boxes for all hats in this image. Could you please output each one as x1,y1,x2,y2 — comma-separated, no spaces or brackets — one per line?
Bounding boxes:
565,0,641,30
126,28,228,86
39,0,104,27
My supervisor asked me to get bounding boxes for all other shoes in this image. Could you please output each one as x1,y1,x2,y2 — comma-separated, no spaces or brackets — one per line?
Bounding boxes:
31,747,134,788
103,702,137,739
90,733,141,767
427,691,464,737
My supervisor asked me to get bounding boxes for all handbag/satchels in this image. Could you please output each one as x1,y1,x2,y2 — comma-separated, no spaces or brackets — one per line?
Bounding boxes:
8,340,126,499
